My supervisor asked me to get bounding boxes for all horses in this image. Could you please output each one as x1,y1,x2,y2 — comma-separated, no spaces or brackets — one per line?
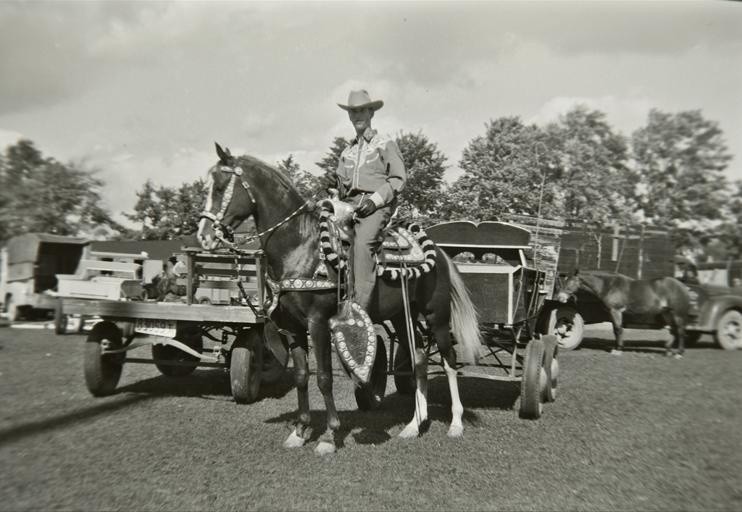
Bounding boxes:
197,140,489,455
557,264,699,358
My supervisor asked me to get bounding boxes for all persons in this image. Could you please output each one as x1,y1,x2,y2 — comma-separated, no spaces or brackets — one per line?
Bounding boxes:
322,90,406,315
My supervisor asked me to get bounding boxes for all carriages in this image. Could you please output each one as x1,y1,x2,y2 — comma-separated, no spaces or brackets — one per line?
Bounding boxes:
196,139,563,456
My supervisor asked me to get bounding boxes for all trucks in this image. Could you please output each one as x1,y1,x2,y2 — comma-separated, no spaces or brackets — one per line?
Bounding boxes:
0,232,92,323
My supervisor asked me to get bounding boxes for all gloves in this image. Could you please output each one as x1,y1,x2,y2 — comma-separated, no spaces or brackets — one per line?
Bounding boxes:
353,199,376,218
322,170,339,190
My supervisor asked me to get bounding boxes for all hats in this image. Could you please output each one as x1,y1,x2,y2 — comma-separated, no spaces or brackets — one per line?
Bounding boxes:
336,89,383,111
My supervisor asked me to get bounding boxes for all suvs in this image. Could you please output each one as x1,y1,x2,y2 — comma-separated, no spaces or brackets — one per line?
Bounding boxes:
671,254,742,352
